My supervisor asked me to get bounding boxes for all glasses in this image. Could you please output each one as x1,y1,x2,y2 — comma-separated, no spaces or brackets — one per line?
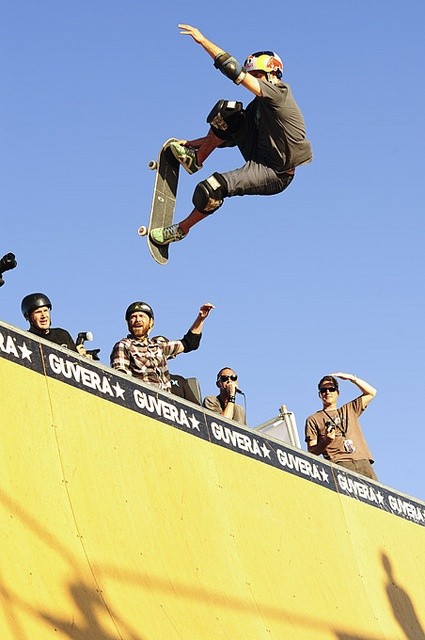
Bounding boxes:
318,388,339,392
217,375,238,382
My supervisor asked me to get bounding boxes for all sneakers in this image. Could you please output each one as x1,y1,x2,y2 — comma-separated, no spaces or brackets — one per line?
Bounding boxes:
149,223,188,246
170,142,203,175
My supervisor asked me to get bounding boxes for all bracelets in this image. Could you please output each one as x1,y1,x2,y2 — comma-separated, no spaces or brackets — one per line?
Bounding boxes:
352,375,357,384
227,395,235,402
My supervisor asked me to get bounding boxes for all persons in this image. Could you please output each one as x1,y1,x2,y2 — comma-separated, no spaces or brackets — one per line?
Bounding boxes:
19,292,93,359
203,367,246,427
305,372,376,479
111,301,215,399
146,23,311,249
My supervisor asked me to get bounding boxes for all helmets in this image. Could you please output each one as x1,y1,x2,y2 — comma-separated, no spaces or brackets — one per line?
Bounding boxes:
125,302,155,319
244,51,283,82
21,293,52,320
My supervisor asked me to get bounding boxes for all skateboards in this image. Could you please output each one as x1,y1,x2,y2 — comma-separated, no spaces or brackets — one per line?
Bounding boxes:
137,138,182,265
